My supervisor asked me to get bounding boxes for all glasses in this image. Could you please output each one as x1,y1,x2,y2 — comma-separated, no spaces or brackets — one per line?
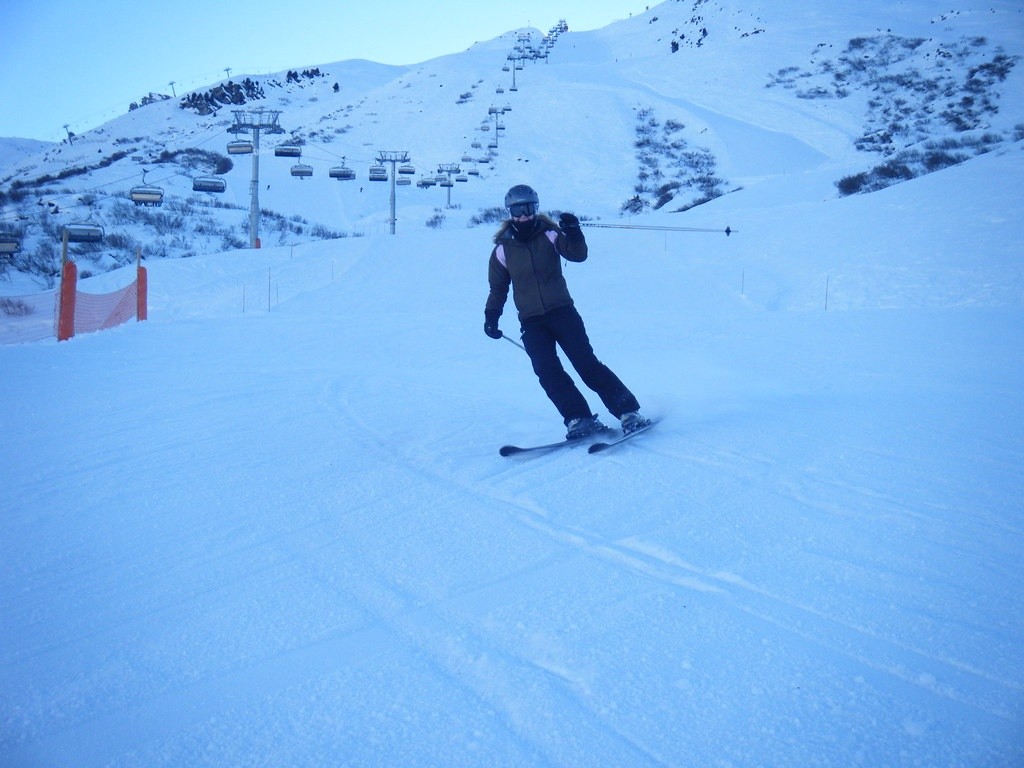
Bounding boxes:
510,202,534,217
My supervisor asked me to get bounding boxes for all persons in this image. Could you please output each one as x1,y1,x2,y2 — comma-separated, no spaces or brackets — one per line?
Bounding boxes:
484,185,644,439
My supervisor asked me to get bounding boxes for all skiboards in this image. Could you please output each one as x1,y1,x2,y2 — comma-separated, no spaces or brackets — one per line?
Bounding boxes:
499,419,664,459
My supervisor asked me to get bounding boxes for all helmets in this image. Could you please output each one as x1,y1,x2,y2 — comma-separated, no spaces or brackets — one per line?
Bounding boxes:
504,185,539,208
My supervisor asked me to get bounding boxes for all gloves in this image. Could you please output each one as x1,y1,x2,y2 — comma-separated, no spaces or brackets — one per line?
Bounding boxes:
484,309,502,339
559,213,583,240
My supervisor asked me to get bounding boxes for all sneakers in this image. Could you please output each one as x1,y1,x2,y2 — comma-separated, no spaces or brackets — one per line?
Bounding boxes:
620,409,645,435
566,412,603,440
